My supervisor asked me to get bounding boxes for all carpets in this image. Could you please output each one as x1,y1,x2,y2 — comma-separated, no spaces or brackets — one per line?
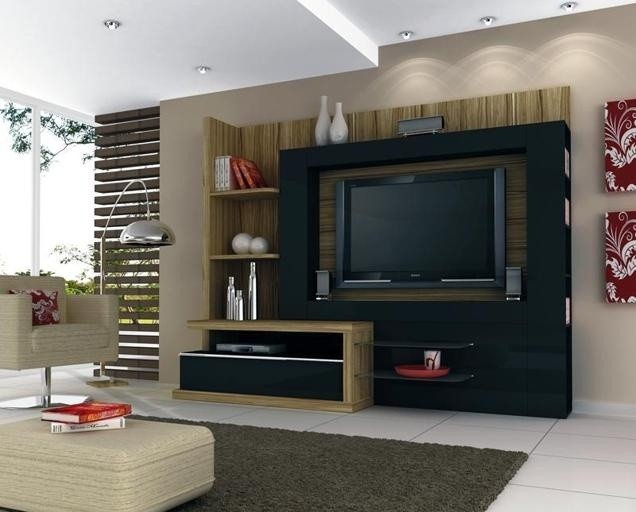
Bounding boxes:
0,415,529,512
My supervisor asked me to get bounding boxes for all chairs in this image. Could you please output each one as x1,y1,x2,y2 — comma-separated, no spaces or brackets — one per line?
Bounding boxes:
0,275,119,410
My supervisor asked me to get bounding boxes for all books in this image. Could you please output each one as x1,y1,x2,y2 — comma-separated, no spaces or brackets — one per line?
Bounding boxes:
214,155,267,192
50,416,126,433
39,399,132,425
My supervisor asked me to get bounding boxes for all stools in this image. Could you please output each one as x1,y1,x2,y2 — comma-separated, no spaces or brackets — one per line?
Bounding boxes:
0,417,216,512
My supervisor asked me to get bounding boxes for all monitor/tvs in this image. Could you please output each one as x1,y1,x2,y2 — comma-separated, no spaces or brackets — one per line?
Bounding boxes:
334,167,506,289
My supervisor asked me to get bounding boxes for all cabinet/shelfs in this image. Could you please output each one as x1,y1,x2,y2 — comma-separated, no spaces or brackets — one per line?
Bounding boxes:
172,86,573,421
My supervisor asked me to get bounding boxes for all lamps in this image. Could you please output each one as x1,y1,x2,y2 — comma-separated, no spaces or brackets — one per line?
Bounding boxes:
85,179,175,389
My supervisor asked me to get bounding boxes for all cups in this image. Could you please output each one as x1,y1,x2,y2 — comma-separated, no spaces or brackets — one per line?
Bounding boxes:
424,350,442,370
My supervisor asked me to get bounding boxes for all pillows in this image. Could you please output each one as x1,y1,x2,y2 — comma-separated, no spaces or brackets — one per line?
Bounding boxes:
7,289,61,325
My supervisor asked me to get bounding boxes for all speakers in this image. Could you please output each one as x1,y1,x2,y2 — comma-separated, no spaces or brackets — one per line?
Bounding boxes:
505,267,523,302
315,270,332,301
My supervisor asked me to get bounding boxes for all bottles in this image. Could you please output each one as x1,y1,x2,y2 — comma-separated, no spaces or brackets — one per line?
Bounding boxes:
226,261,256,321
